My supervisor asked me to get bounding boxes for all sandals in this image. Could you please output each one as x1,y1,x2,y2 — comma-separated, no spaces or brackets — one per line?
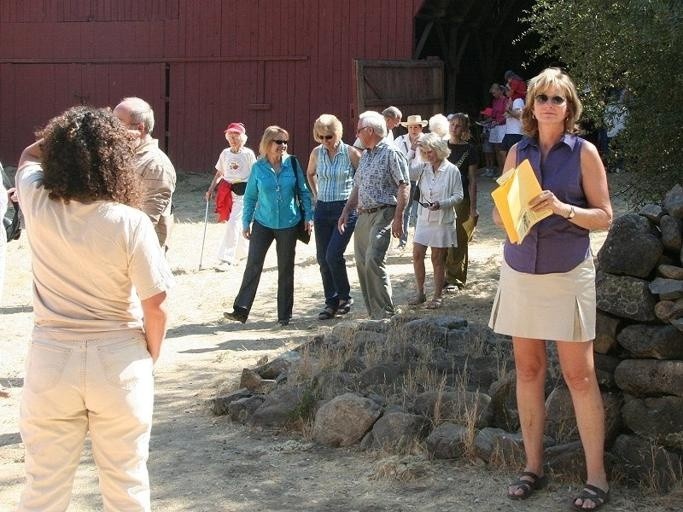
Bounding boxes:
318,304,336,320
429,298,444,310
408,295,427,307
445,283,461,295
336,297,355,314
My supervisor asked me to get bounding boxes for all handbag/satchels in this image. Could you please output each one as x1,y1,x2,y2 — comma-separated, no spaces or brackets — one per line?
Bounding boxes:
231,182,247,196
295,206,311,245
2,212,22,244
412,185,421,201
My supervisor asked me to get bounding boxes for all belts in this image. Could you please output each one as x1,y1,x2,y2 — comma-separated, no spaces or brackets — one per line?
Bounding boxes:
355,204,396,214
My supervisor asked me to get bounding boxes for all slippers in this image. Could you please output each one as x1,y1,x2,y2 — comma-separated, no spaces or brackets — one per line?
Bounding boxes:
569,484,612,511
506,469,551,501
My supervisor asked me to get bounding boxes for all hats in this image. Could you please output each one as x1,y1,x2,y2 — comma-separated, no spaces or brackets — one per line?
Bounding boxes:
399,114,429,129
479,107,493,117
224,122,246,134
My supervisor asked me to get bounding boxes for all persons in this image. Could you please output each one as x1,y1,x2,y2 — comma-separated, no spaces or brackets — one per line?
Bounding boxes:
306,112,363,321
207,123,256,271
407,113,480,309
482,70,632,172
14,101,168,512
491,65,614,512
112,96,178,257
224,124,313,325
355,106,450,249
0,174,19,399
337,110,412,320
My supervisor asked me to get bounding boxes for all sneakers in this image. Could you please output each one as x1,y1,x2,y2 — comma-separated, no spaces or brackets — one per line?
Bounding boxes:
214,260,235,273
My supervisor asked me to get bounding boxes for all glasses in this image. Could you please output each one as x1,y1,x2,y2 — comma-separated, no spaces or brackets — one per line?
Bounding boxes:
271,139,288,144
534,93,568,107
354,126,370,134
317,134,334,140
415,196,434,209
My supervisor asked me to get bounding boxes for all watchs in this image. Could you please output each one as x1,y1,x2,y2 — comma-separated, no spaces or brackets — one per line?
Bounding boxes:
566,204,575,220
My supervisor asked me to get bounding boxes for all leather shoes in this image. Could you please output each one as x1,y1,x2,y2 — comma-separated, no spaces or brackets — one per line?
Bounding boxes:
276,310,293,328
222,306,251,325
